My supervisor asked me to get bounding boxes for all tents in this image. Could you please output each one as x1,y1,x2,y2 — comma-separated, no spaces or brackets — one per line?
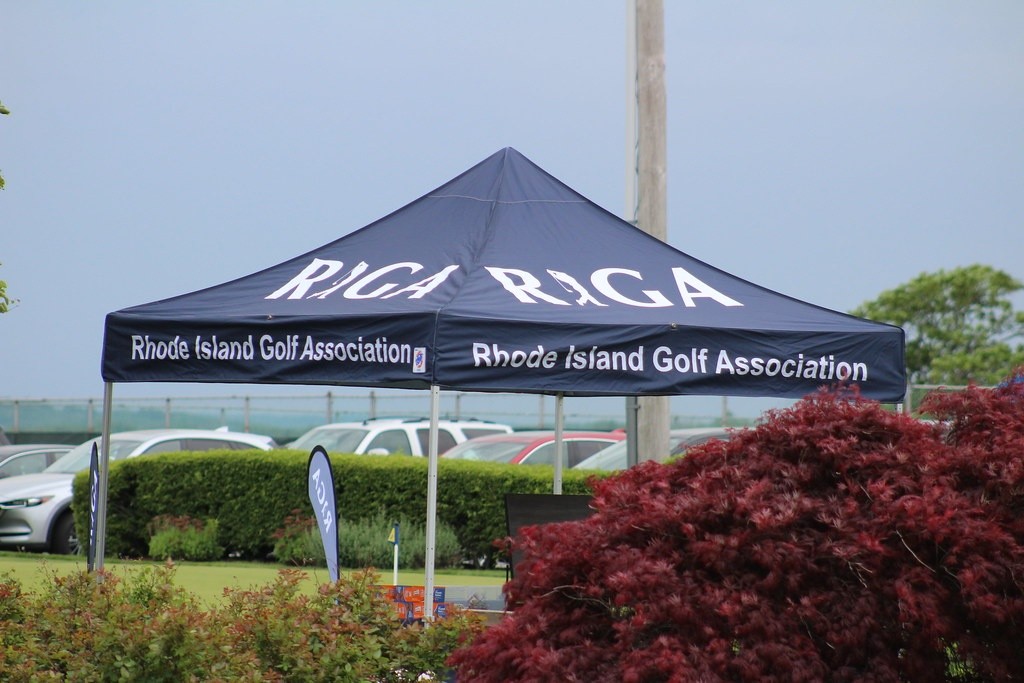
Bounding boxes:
96,147,907,629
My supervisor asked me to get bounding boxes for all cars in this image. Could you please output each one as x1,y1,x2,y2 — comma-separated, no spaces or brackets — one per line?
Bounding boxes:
0,444,79,482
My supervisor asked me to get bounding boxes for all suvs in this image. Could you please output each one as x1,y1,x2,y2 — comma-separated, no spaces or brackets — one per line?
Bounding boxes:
437,427,627,470
565,425,753,471
280,413,514,456
0,423,282,556
